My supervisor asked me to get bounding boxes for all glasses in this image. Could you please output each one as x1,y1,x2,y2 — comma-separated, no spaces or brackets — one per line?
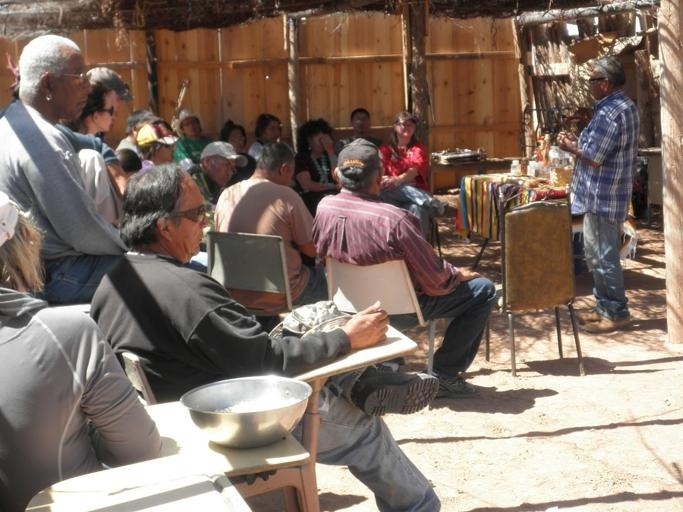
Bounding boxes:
168,204,204,223
98,107,113,116
57,71,91,85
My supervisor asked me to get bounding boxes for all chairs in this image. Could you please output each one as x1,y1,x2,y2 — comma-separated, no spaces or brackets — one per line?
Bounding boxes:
326,257,445,375
485,201,586,377
206,231,292,316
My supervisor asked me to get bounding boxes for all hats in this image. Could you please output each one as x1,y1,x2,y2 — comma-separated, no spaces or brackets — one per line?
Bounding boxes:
394,111,419,124
1,190,20,249
201,142,248,166
338,139,380,180
136,123,178,148
175,109,204,137
300,119,332,138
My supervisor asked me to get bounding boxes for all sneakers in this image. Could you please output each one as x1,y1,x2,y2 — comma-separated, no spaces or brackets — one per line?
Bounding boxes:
350,369,478,416
578,312,631,334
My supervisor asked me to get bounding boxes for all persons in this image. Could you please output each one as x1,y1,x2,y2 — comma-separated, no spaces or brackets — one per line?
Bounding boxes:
555,55,641,332
1,34,498,511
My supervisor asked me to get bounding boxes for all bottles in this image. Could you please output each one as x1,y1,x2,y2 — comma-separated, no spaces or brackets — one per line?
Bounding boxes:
511,160,536,176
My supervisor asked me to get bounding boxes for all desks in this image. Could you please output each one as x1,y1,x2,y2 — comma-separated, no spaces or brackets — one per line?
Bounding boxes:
454,173,636,274
102,401,320,512
292,323,417,464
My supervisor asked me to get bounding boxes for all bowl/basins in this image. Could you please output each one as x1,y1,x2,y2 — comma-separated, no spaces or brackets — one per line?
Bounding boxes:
180,376,313,450
